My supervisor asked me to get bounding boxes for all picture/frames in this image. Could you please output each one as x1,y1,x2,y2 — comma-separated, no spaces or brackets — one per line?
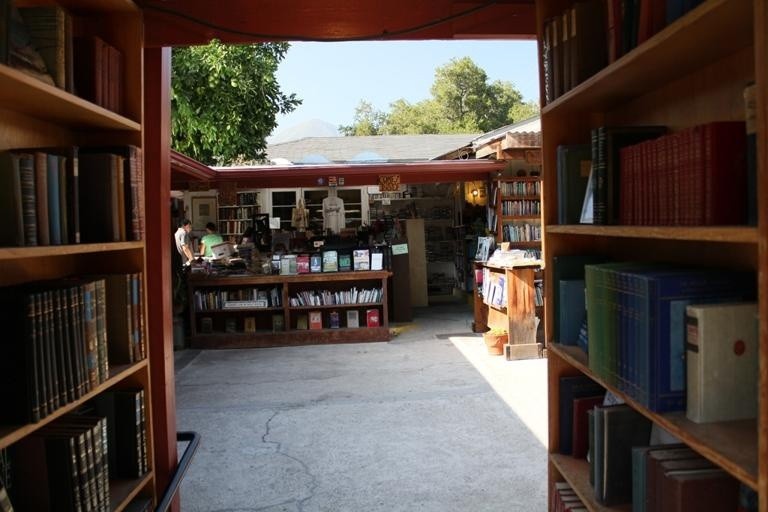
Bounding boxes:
191,196,217,233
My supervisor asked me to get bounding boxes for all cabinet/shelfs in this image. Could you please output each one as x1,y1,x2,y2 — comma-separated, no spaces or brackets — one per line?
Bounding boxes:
474,174,544,359
538,2,768,449
3,0,156,512
186,271,392,347
219,188,372,246
375,185,469,319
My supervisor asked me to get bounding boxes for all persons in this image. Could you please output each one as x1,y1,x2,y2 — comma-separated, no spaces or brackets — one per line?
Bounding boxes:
238,226,254,246
199,222,223,257
174,218,194,266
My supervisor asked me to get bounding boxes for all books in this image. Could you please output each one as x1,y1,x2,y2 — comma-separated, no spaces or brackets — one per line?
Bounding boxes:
1,388,147,511
2,2,123,114
557,81,756,228
2,144,144,247
192,244,383,332
551,377,739,510
2,272,145,423
474,182,541,307
553,253,756,424
218,192,261,233
542,2,700,106
